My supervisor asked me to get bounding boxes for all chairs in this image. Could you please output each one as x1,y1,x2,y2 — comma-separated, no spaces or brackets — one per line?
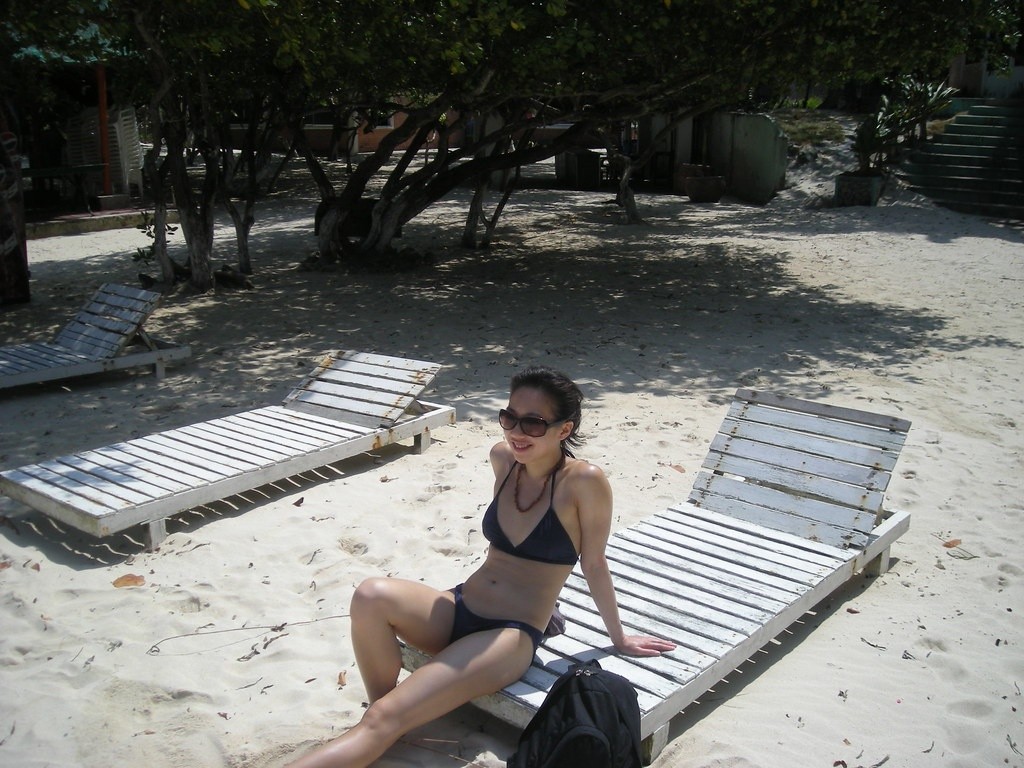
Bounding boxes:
0,283,192,388
0,350,457,553
397,388,911,766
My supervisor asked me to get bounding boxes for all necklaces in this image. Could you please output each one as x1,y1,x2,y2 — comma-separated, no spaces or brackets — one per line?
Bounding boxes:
514,457,563,513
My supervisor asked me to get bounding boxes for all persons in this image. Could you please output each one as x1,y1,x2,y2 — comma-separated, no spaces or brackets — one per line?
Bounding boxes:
464,117,474,146
284,368,676,768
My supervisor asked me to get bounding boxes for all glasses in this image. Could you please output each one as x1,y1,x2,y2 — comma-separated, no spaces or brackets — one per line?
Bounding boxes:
499,410,566,438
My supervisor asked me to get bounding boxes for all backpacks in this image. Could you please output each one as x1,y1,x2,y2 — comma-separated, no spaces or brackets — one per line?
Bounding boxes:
507,659,642,768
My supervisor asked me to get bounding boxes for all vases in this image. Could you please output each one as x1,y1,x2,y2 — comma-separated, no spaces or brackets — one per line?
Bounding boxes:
685,175,726,202
